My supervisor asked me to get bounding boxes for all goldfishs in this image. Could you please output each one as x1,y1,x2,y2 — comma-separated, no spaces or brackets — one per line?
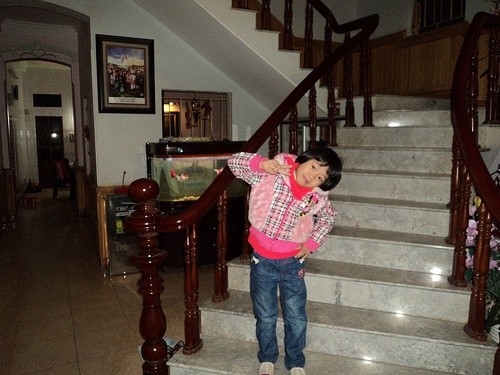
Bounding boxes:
170,168,189,182
213,168,224,175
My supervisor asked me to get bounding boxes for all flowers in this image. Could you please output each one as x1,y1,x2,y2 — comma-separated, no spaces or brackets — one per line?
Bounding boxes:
465,195,481,271
490,224,500,272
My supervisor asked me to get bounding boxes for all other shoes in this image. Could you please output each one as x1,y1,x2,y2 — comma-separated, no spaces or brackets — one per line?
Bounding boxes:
290,367,306,375
259,362,274,375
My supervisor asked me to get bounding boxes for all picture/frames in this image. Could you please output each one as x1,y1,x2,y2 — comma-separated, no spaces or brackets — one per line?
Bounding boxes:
94,33,157,114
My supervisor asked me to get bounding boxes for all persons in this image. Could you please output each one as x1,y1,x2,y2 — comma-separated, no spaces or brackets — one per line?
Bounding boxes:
228,146,343,375
